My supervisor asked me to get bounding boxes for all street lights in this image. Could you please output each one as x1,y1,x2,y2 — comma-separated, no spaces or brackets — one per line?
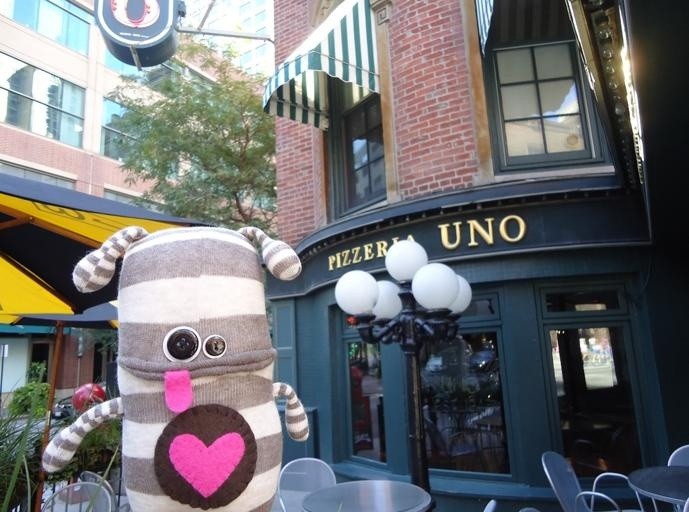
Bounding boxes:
334,239,473,511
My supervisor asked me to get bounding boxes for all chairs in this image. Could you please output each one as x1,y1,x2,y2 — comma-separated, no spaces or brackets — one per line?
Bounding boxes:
277,457,337,512
41,471,117,511
542,446,689,512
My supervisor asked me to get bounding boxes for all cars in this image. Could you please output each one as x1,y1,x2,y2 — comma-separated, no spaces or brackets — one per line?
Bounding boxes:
51,382,107,421
418,331,502,419
349,355,382,430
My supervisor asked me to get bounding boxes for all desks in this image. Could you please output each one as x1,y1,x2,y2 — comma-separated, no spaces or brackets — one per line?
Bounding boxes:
303,479,431,512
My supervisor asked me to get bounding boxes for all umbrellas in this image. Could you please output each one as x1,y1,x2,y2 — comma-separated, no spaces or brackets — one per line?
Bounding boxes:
0,175,216,314
1,302,118,511
